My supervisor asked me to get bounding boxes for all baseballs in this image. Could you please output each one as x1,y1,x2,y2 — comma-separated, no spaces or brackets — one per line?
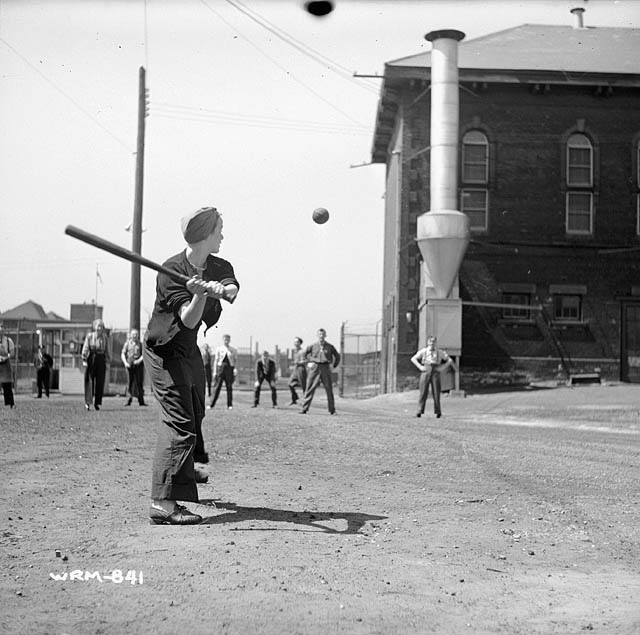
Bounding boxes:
313,208,329,224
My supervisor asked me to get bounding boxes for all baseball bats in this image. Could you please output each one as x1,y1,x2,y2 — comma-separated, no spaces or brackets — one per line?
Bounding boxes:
64,224,237,304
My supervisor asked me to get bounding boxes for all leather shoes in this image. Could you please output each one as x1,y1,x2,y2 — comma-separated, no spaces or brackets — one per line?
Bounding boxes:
149,504,202,525
194,469,208,483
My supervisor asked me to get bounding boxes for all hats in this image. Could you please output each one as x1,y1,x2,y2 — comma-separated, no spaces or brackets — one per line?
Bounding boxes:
180,206,218,243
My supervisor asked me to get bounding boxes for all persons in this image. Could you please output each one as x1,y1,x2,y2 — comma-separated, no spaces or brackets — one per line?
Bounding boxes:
82,320,111,408
300,329,340,416
35,345,53,398
252,350,280,410
288,337,309,405
199,343,212,396
144,205,239,523
207,333,237,410
410,335,452,418
121,328,149,406
0,327,16,407
